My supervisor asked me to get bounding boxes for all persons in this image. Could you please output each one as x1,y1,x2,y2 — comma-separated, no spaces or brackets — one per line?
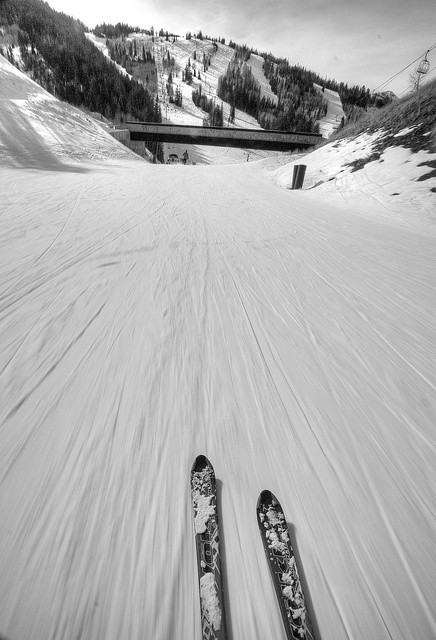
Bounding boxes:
182,150,188,165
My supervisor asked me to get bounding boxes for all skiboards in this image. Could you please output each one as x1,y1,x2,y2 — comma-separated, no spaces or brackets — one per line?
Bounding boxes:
190,454,313,639
